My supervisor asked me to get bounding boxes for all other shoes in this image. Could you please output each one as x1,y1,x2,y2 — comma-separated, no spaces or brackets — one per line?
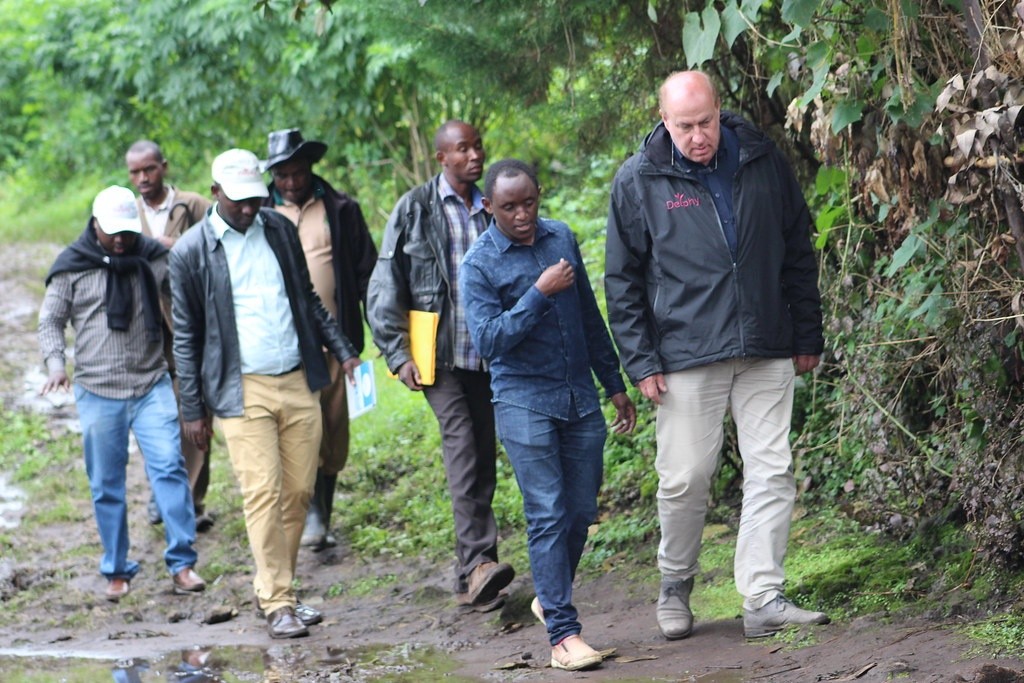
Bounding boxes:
194,503,214,528
106,578,129,600
173,568,206,596
148,492,162,525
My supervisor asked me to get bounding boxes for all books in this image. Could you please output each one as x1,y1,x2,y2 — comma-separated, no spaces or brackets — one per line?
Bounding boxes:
343,360,377,422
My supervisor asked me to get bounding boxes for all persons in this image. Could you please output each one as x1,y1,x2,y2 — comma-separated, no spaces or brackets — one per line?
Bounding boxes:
364,120,638,671
604,71,830,639
36,127,379,638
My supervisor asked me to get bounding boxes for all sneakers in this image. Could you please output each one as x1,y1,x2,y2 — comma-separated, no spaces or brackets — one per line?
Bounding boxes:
465,561,515,604
551,634,603,670
457,591,510,616
267,361,300,376
656,576,695,640
531,596,547,625
742,591,831,637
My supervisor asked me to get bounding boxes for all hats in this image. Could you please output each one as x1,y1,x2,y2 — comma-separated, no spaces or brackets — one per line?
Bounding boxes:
92,185,142,234
265,128,328,171
211,148,269,201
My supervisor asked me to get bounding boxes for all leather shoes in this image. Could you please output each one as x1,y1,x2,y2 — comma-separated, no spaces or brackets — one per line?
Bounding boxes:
255,596,322,639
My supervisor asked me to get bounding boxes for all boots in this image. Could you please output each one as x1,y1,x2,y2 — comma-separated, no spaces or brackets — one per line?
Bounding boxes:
298,466,338,554
